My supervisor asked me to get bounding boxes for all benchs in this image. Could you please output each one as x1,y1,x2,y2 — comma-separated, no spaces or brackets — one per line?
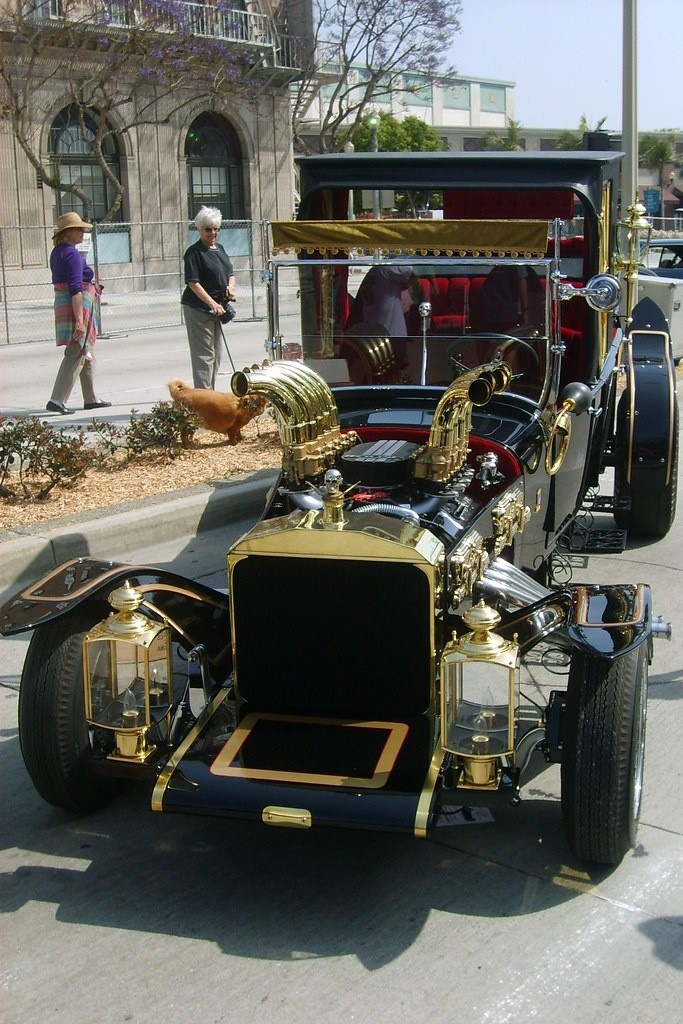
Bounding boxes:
408,272,619,389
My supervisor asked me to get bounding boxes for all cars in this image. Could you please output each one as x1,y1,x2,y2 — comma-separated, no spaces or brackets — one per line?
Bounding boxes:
0,150,679,867
638,239,683,278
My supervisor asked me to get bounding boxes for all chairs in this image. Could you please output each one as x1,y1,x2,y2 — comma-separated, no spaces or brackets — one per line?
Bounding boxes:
475,264,549,342
340,260,432,342
441,333,539,444
659,259,673,268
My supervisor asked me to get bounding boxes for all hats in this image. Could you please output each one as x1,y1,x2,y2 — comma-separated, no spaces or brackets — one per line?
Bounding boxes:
51,211,94,239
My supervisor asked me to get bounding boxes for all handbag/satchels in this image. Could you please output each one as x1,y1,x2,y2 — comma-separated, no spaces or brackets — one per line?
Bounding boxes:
216,299,234,324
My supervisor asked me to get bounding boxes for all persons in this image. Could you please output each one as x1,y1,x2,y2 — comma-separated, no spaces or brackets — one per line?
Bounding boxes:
471,265,529,380
360,264,415,372
182,206,236,389
46,212,112,415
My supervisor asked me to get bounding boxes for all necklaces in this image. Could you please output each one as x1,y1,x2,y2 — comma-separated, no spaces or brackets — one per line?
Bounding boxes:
199,239,218,250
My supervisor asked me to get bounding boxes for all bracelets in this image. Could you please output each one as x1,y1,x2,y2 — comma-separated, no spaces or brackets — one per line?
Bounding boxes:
521,307,528,311
229,285,234,289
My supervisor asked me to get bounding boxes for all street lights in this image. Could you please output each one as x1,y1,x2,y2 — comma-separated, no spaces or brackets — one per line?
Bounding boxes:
343,140,357,220
368,113,382,259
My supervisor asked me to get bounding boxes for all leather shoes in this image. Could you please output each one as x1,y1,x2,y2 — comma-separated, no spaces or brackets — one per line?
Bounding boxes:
84,399,112,409
46,401,75,415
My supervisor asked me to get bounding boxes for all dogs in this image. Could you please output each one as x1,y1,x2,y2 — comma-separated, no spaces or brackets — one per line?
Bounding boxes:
168,377,271,448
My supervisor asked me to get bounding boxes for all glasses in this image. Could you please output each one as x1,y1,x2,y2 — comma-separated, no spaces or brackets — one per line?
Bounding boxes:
199,227,220,232
